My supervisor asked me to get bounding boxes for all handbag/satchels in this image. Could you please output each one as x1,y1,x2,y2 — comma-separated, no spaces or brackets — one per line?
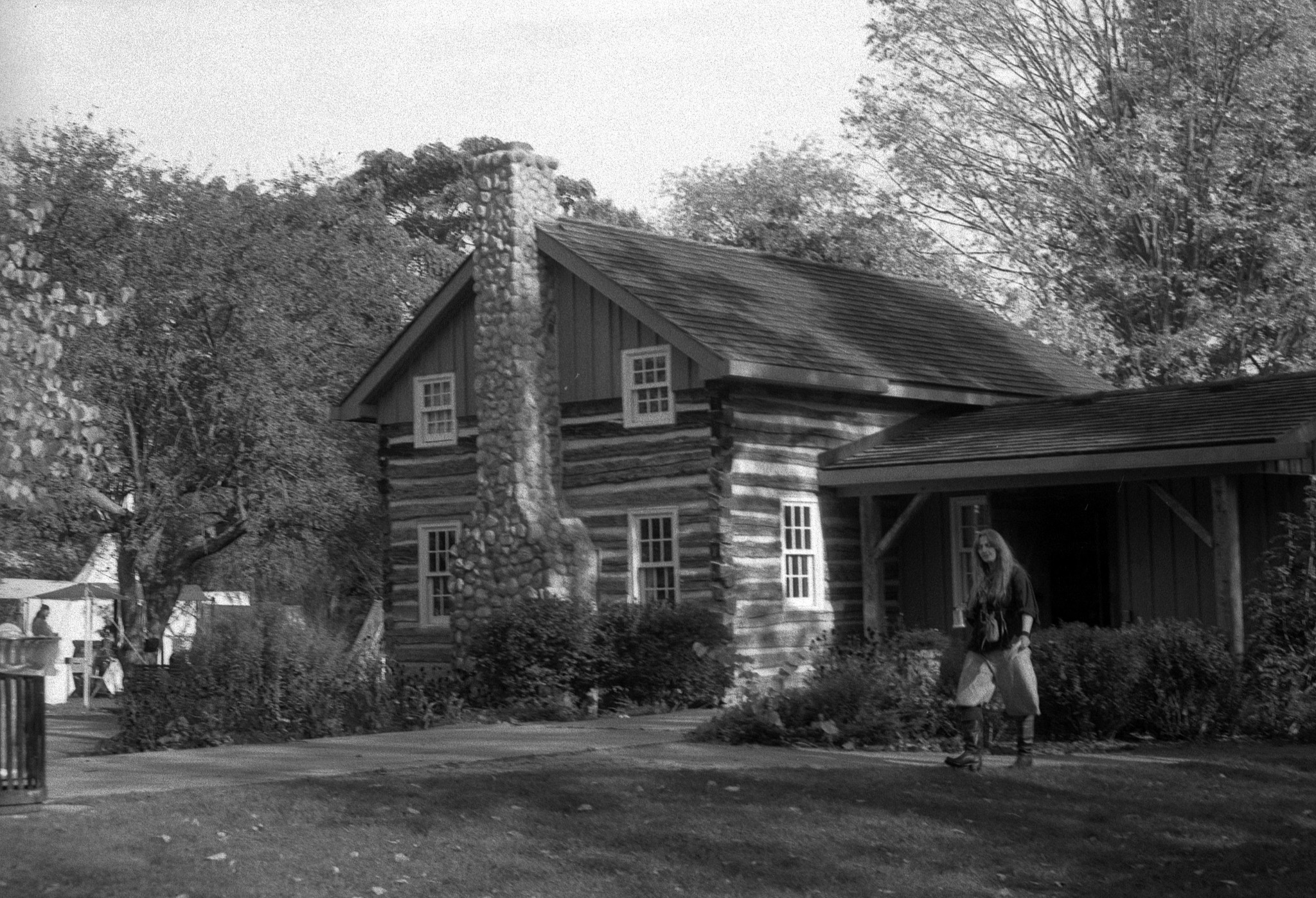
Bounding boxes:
979,612,999,649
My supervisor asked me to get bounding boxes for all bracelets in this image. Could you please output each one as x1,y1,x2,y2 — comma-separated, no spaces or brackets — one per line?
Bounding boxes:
1021,631,1030,637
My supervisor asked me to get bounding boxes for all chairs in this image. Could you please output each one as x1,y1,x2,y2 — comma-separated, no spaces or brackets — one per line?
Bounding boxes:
91,658,121,698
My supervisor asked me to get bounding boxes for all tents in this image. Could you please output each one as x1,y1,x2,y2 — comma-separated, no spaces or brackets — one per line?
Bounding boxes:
27,582,215,707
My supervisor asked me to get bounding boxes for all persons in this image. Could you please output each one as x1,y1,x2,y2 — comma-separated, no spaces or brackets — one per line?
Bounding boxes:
945,529,1040,770
97,625,125,699
32,604,58,638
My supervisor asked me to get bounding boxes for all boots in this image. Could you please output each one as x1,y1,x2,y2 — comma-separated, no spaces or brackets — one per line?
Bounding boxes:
944,719,982,770
1003,719,1033,768
